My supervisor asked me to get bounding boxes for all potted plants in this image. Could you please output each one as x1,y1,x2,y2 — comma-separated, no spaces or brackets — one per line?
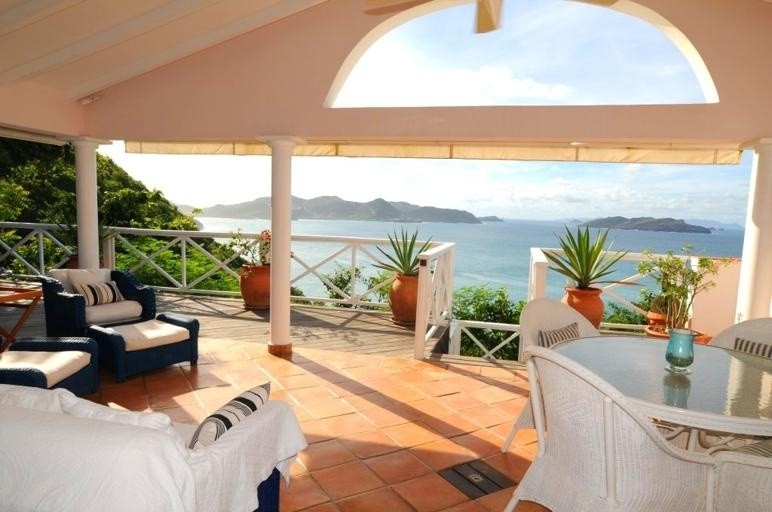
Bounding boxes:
636,244,736,345
540,223,646,328
370,224,433,326
646,295,680,326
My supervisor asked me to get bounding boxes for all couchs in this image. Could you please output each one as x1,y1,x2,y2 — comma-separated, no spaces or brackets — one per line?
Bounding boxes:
37,267,156,337
87,312,200,385
0,336,102,397
1,385,308,512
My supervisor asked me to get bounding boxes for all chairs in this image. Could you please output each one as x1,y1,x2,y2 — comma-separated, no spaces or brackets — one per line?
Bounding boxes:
500,298,603,453
717,436,772,512
501,346,717,512
705,317,771,456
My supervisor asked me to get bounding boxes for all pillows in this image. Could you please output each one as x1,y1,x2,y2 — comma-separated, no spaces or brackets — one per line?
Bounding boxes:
59,392,176,436
72,281,125,305
188,381,271,449
0,385,62,414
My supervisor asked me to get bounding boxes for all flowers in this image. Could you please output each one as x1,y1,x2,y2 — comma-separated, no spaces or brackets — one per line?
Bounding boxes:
228,227,272,266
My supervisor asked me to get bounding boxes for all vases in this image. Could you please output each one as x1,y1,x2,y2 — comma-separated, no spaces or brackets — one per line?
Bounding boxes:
239,264,271,310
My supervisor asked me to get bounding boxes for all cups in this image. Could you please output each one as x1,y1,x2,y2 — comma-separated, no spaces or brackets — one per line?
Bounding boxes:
662,373,690,409
665,328,699,375
